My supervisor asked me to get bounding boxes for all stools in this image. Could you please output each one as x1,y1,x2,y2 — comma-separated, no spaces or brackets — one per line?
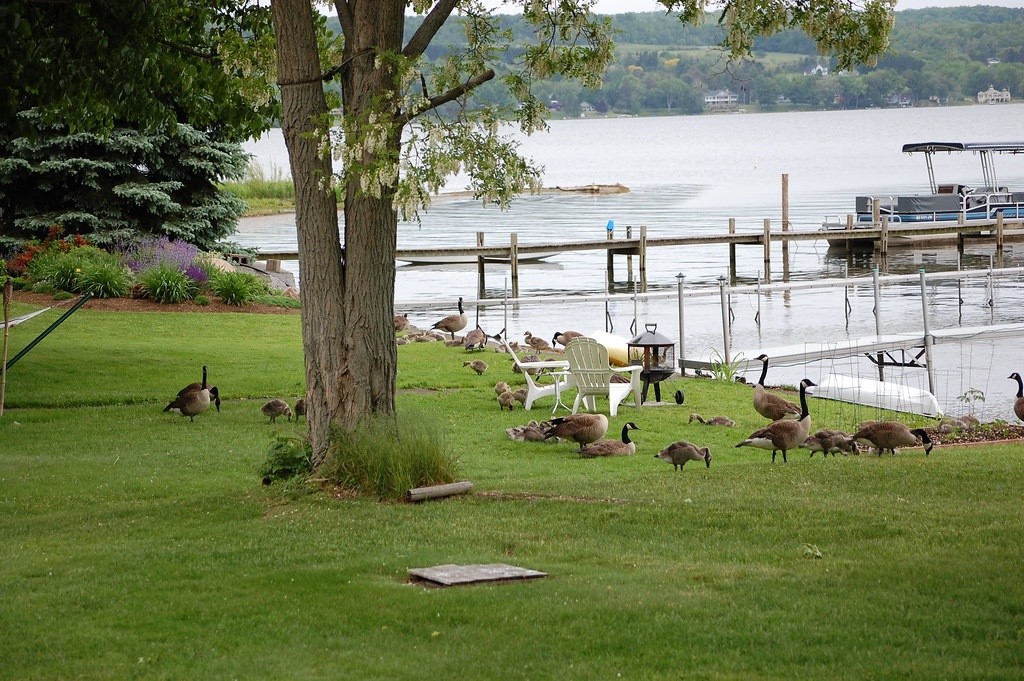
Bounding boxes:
542,371,572,415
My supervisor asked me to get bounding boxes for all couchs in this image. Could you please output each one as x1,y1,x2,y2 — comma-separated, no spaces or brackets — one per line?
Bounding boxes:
969,187,1008,206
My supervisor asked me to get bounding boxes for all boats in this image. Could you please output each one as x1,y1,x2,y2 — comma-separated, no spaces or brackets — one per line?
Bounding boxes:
811,373,944,420
820,140,1024,246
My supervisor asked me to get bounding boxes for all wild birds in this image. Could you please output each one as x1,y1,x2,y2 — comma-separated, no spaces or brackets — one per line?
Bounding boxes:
653,441,712,472
430,297,468,340
1007,373,1024,422
798,431,863,458
511,389,535,406
735,379,818,463
753,354,801,421
573,422,642,457
496,392,515,411
552,331,583,348
394,313,409,332
853,421,933,459
463,360,489,375
505,412,608,449
688,413,736,427
494,382,513,395
162,365,306,424
508,356,557,375
464,329,488,352
495,331,549,354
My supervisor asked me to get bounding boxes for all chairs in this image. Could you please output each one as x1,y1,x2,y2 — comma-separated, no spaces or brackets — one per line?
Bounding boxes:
499,334,589,411
565,336,643,417
